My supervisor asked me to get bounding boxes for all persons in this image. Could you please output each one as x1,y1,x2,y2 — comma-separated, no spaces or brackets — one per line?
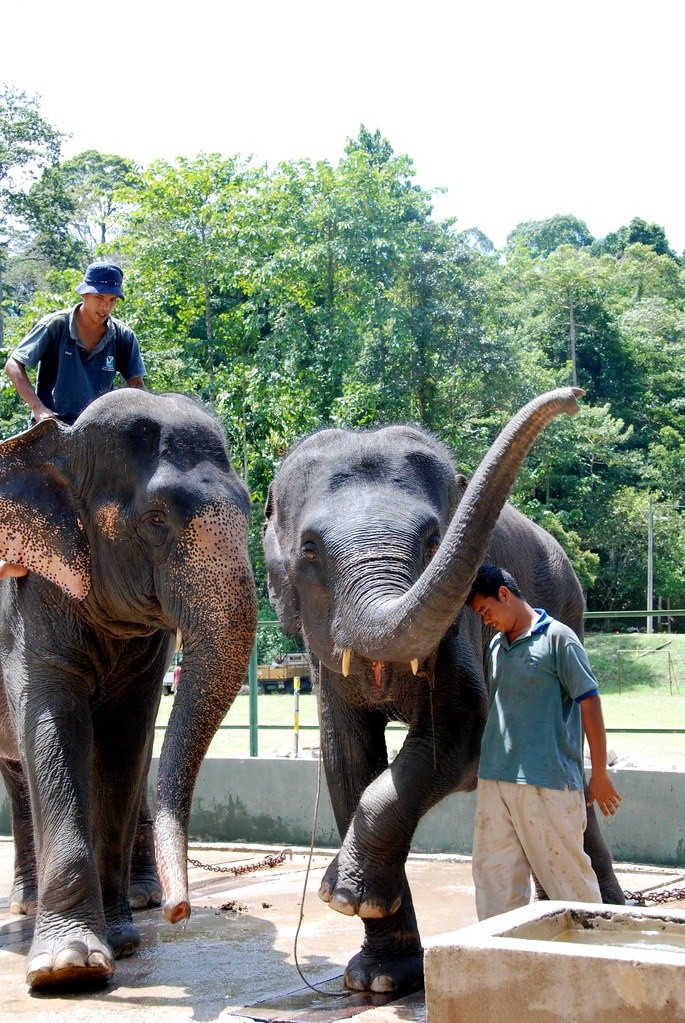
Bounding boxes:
171,661,180,696
4,262,147,424
269,655,287,670
464,563,623,922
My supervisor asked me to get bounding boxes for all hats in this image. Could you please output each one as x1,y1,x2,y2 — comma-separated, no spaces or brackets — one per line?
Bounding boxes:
74,261,124,302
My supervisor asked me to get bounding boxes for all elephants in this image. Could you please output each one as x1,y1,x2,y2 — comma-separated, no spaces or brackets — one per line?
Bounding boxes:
0,386,256,990
259,382,628,995
